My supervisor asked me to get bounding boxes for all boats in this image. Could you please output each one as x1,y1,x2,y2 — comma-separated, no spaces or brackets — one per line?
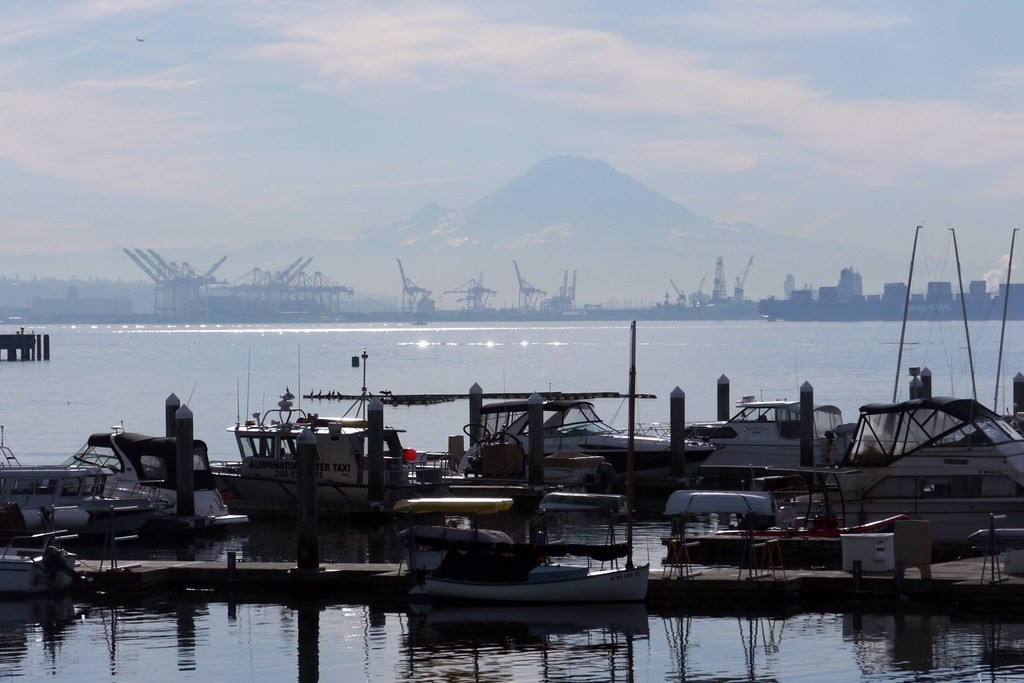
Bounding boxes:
447,398,717,480
0,462,167,544
658,489,913,564
681,392,860,470
401,602,655,662
735,397,1023,545
406,541,653,602
63,434,250,527
209,414,583,515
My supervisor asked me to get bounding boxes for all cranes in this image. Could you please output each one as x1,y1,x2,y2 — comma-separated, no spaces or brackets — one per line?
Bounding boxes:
122,247,761,311
